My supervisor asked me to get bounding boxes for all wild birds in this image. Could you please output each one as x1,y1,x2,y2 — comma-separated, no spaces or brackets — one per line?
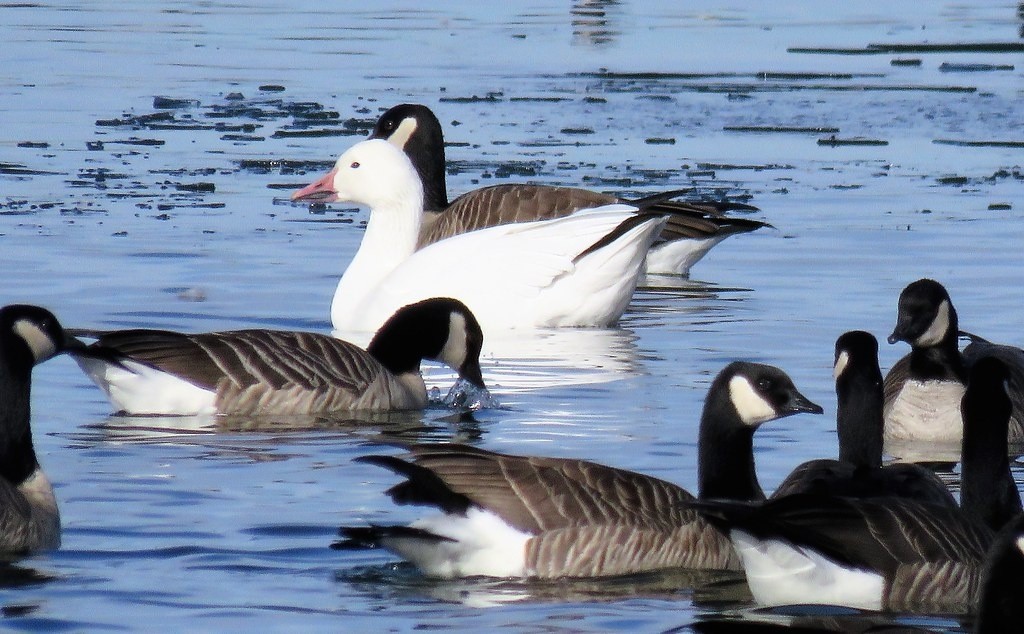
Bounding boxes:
1,102,1023,633
